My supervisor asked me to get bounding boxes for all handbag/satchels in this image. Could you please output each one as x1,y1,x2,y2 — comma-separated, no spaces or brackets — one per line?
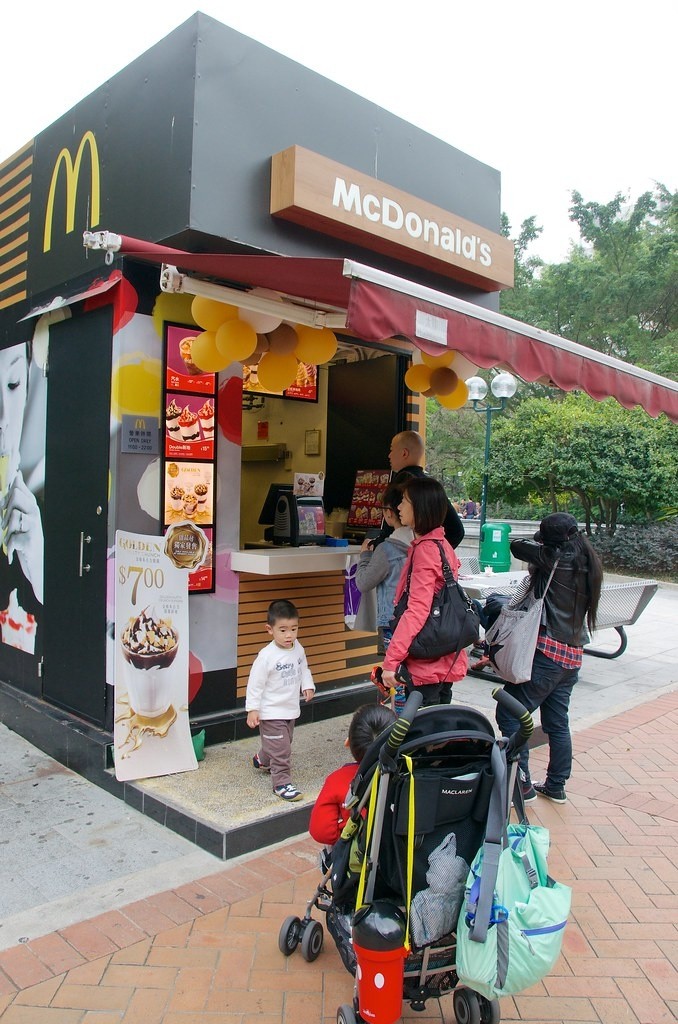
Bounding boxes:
388,540,479,659
484,555,560,684
455,824,573,1001
344,553,377,632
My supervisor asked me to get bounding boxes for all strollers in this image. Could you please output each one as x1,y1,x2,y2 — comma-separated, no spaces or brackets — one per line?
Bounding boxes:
276,686,535,1024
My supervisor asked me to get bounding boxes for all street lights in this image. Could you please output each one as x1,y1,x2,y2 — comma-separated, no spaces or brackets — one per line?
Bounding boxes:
464,371,517,570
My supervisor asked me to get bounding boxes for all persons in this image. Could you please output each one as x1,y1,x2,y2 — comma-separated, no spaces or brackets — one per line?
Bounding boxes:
367,430,465,551
354,484,415,713
0,341,43,657
494,513,602,805
380,476,469,707
245,600,316,801
449,496,481,519
308,703,398,876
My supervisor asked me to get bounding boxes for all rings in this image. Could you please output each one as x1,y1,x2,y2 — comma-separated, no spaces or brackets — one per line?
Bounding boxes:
19,512,23,531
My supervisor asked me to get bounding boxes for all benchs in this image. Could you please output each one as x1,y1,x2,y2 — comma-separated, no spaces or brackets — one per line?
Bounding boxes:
472,569,659,682
457,557,480,581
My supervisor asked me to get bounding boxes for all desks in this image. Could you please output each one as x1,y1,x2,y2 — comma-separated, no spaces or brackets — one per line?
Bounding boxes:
466,572,527,601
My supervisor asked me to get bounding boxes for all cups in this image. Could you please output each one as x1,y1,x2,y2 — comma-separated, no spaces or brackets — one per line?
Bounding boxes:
178,420,201,441
183,499,199,519
194,490,208,506
199,416,214,437
166,408,181,440
353,473,389,519
242,365,258,389
120,630,180,717
170,494,184,510
0,610,37,656
297,374,317,387
179,336,202,375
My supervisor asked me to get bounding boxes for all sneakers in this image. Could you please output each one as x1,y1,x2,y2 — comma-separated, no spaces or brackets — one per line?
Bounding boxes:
511,786,537,806
273,783,303,801
253,754,271,773
531,781,567,803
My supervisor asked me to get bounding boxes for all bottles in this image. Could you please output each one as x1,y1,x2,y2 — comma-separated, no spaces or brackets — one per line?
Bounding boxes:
349,902,410,1023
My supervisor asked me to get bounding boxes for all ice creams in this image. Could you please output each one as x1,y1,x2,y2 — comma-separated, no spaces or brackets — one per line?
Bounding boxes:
165,399,215,442
352,489,377,520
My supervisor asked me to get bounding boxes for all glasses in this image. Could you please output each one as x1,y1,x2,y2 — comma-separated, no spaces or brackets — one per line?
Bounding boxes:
378,506,396,513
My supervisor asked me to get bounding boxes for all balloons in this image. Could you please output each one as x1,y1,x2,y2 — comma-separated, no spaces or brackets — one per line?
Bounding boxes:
191,295,338,393
404,346,480,410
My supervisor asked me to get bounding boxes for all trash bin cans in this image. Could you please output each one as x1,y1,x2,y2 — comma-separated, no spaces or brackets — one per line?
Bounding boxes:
478,523,511,573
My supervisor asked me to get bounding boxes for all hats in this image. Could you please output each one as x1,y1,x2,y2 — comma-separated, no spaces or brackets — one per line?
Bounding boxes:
534,512,586,543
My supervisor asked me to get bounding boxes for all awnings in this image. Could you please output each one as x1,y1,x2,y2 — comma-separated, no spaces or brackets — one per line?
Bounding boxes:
119,234,678,424
